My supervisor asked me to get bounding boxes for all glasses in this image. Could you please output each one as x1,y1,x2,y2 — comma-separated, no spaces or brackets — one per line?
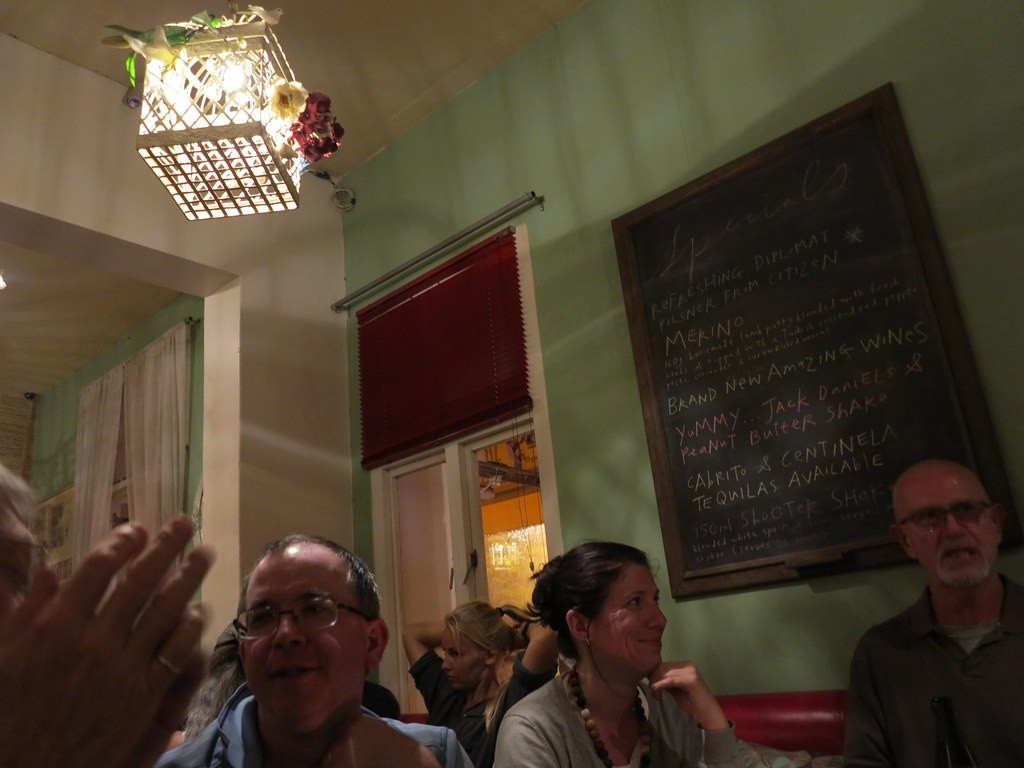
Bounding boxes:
232,595,373,640
898,500,991,530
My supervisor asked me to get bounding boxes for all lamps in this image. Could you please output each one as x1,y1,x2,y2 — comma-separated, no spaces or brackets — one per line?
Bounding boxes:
135,20,301,222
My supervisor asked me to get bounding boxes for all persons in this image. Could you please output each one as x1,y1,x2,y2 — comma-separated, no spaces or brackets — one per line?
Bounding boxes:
402,601,560,768
843,459,1024,768
0,463,217,768
493,542,747,768
154,533,475,768
363,681,401,722
163,618,246,754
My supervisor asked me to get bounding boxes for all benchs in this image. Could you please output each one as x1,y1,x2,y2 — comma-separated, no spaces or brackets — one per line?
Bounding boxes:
715,691,846,752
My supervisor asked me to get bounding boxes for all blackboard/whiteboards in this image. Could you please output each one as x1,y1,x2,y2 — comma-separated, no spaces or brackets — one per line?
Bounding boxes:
610,81,1023,600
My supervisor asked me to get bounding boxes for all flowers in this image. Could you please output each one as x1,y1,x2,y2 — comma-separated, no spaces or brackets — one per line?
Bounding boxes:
103,4,346,163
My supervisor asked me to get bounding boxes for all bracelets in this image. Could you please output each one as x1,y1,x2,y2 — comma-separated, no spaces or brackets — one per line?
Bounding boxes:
521,622,532,641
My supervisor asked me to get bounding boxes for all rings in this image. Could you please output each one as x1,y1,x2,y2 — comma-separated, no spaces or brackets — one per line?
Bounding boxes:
158,653,181,676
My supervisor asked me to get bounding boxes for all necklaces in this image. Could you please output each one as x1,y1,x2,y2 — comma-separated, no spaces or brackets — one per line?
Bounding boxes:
568,667,652,768
